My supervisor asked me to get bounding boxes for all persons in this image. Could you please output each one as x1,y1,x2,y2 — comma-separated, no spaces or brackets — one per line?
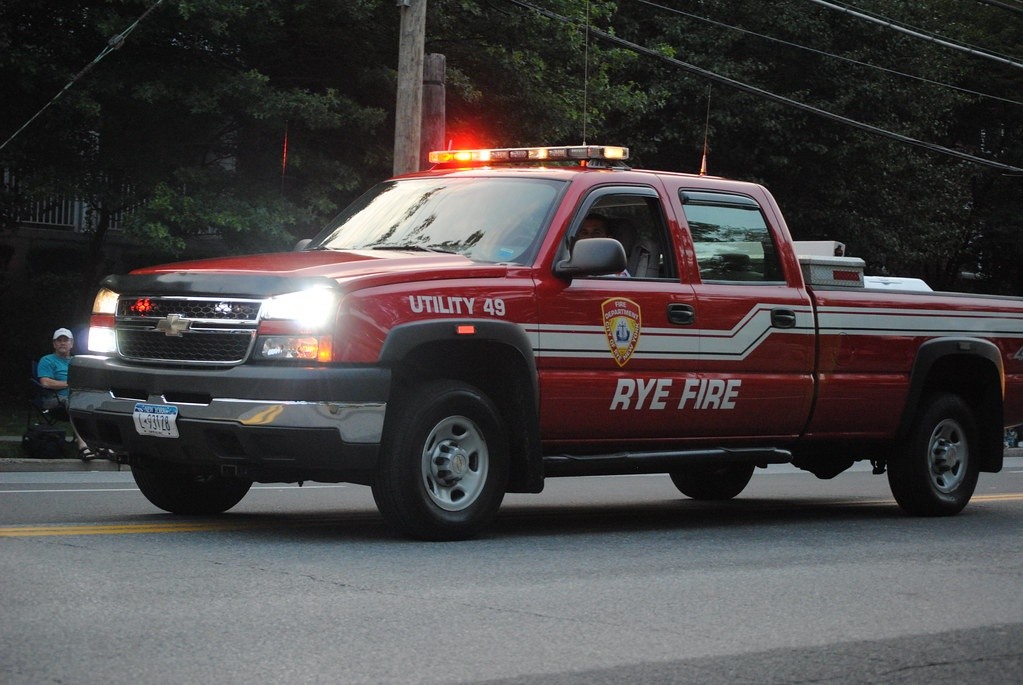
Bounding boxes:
37,328,120,463
579,213,632,279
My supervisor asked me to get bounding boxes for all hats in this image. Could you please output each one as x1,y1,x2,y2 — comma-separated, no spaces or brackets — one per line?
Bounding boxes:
52,327,74,341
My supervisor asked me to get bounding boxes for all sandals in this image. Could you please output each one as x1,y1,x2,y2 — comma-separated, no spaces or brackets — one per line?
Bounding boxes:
78,446,96,460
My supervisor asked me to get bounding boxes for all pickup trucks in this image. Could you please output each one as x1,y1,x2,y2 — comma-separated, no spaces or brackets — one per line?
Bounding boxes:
66,144,1023,541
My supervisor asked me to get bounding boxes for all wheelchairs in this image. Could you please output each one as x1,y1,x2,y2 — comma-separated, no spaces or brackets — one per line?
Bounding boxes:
21,363,80,459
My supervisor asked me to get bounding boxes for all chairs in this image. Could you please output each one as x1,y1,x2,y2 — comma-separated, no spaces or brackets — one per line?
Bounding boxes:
610,220,659,277
23,356,118,462
